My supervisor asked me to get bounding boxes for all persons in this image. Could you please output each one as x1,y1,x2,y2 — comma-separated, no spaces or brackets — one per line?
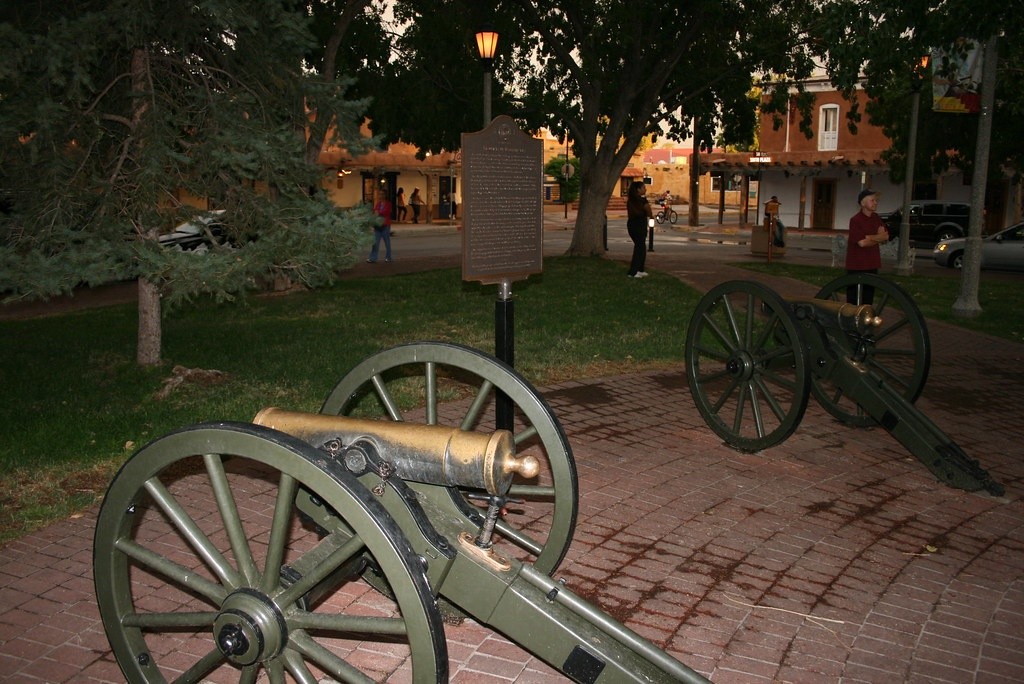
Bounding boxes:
397,187,407,222
846,190,887,304
765,196,786,247
661,190,673,220
628,181,652,278
448,191,457,220
409,187,424,224
366,189,392,263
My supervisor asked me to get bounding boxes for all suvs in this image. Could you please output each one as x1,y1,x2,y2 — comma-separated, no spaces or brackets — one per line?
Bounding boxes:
931,244,931,246
875,198,987,245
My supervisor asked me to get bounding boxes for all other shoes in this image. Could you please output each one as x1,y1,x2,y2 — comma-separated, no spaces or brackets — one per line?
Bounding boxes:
628,274,642,277
637,271,648,277
386,258,392,262
367,258,376,263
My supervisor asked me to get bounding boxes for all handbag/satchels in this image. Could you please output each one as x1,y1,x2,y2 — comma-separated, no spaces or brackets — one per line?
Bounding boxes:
408,197,412,204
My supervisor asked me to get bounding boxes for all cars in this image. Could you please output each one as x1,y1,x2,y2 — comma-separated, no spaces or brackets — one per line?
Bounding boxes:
157,209,261,254
931,221,1024,273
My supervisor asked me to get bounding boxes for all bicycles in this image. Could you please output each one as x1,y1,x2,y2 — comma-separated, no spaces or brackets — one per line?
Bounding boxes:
655,199,678,225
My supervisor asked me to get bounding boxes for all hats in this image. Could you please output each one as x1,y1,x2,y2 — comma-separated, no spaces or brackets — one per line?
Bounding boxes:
858,190,875,205
